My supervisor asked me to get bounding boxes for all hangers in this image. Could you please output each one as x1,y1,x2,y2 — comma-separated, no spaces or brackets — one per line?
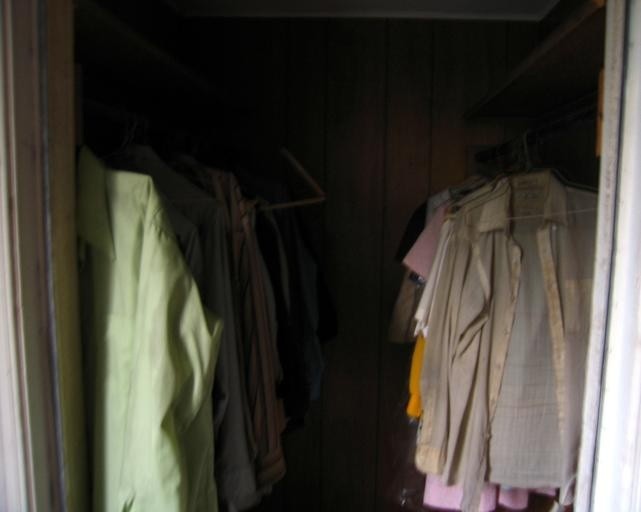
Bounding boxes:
441,123,598,231
104,109,327,214
